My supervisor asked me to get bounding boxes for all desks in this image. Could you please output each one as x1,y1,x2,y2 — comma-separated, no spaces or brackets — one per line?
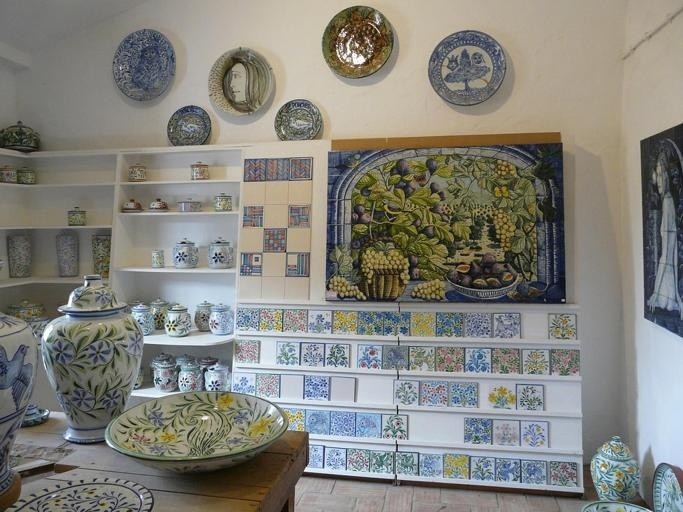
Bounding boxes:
0,412,308,512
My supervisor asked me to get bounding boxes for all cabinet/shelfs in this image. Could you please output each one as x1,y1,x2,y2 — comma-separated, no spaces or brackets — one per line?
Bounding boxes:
0,147,115,320
232,132,584,493
110,143,245,399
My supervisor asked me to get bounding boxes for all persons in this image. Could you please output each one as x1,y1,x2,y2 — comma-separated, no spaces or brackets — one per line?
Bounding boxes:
230,53,267,113
647,153,682,314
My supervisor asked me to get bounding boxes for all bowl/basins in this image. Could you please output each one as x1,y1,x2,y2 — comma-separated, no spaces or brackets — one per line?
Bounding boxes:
652,463,683,511
104,390,289,473
446,273,520,300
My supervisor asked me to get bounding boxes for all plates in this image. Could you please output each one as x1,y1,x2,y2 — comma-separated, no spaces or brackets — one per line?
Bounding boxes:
322,5,394,79
274,98,323,141
145,209,168,212
208,48,274,117
112,29,176,101
167,105,211,146
582,500,654,512
122,210,144,212
428,30,506,106
4,478,153,512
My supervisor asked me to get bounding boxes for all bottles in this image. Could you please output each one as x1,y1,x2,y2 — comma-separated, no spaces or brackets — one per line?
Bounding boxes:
190,160,209,180
177,192,233,212
127,162,147,182
128,296,235,337
133,352,232,392
68,207,87,226
0,275,144,512
0,165,37,184
91,229,112,278
151,236,234,268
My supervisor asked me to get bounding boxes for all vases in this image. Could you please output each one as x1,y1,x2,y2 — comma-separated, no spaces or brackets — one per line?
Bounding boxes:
92,235,111,279
